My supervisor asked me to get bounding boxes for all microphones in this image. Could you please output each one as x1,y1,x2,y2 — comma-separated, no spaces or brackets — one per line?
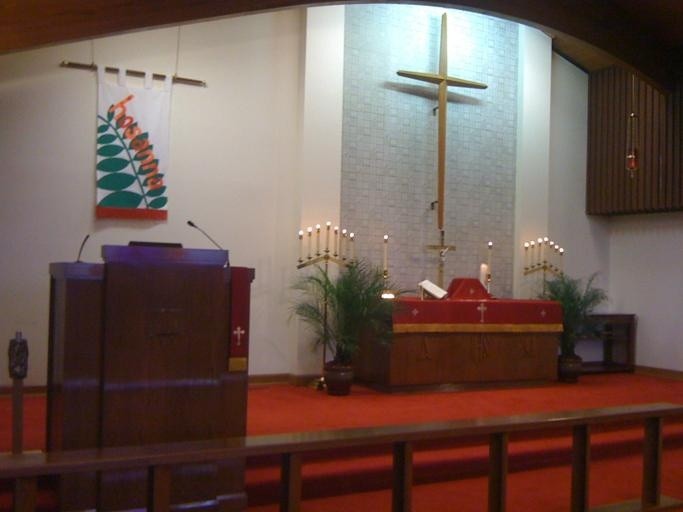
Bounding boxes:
187,220,230,267
75,234,90,263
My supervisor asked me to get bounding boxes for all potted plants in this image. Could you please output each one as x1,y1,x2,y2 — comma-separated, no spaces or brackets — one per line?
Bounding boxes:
532,266,612,384
285,253,402,395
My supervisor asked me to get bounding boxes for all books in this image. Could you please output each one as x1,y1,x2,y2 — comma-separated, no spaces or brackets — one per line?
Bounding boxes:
417,279,448,299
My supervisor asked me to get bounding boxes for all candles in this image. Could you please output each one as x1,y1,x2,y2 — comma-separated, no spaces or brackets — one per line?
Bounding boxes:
296,221,361,267
520,235,567,279
484,241,494,279
381,232,389,272
